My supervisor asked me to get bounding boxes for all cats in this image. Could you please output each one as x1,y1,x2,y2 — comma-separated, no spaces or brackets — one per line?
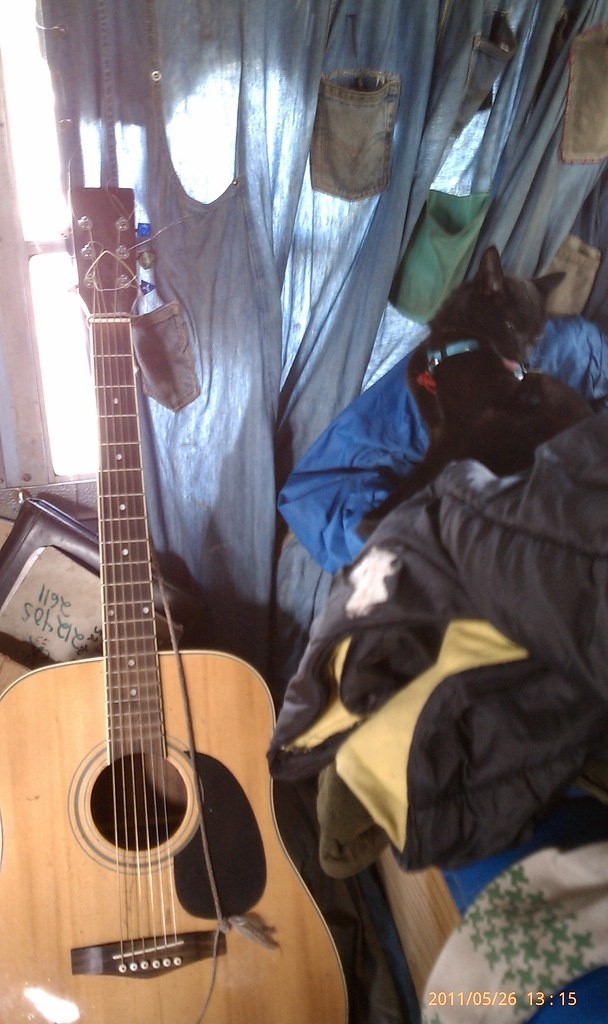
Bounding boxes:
351,245,592,545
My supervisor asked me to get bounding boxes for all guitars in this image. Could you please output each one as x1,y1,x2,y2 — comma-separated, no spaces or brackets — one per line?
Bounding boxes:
0,185,351,1024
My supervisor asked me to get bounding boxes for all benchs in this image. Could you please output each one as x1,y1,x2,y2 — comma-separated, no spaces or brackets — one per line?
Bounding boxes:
369,781,607,1023
